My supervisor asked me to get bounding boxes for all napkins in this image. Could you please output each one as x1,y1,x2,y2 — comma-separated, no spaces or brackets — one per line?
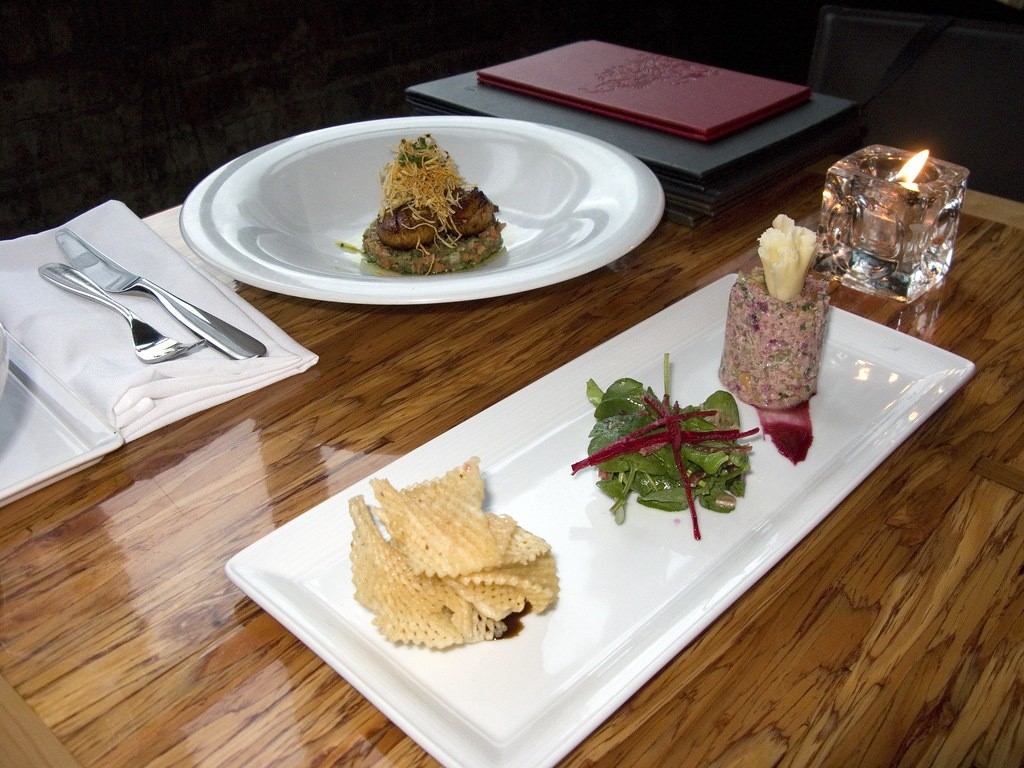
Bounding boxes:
0,198,321,447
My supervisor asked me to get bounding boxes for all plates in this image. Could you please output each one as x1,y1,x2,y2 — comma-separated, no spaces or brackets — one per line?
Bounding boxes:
179,116,666,305
224,274,976,768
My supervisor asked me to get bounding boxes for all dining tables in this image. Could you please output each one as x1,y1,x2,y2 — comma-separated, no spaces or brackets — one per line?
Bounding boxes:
0,148,1023,768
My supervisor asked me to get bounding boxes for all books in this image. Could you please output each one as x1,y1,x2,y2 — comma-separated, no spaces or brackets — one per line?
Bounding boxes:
405,38,872,230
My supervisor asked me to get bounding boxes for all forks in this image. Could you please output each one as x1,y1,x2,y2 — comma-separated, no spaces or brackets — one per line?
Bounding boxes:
41,262,208,364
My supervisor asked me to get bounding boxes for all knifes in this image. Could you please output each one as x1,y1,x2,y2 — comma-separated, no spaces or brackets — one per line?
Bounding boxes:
55,227,269,361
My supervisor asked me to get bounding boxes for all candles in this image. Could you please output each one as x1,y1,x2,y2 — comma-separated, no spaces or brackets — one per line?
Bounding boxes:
884,148,934,187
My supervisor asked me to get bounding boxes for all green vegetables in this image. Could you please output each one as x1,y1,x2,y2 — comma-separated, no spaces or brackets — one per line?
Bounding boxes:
585,351,751,525
